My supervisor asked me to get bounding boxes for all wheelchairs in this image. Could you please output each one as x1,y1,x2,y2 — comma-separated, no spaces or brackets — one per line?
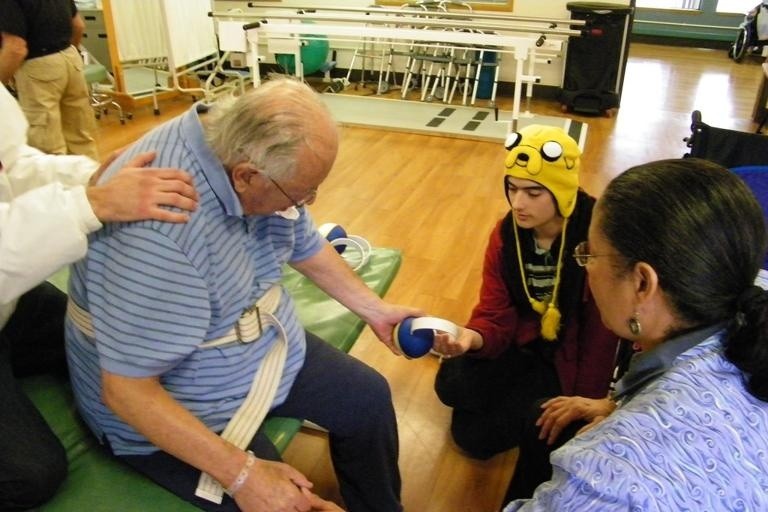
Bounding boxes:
732,3,767,61
611,109,768,401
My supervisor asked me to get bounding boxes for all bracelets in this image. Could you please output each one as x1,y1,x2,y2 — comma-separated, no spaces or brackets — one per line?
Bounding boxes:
220,449,257,500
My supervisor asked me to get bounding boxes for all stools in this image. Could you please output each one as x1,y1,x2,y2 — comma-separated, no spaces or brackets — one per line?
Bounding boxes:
83,62,125,126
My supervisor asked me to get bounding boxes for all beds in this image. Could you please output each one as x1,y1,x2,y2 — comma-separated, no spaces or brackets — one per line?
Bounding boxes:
0,245,404,512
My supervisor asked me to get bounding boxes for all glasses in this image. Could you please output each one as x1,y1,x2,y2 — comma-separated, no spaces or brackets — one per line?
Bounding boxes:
574,242,625,268
239,148,315,208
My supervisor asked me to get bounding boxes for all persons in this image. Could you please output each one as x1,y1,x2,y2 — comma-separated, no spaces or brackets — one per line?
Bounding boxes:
500,156,768,512
422,124,633,462
60,67,433,512
0,1,100,167
0,80,201,512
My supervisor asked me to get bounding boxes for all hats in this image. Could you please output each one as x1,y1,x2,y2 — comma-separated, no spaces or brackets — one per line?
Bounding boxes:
502,124,582,219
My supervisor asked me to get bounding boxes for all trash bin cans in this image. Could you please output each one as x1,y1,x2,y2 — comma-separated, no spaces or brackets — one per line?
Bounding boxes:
561,2,635,118
